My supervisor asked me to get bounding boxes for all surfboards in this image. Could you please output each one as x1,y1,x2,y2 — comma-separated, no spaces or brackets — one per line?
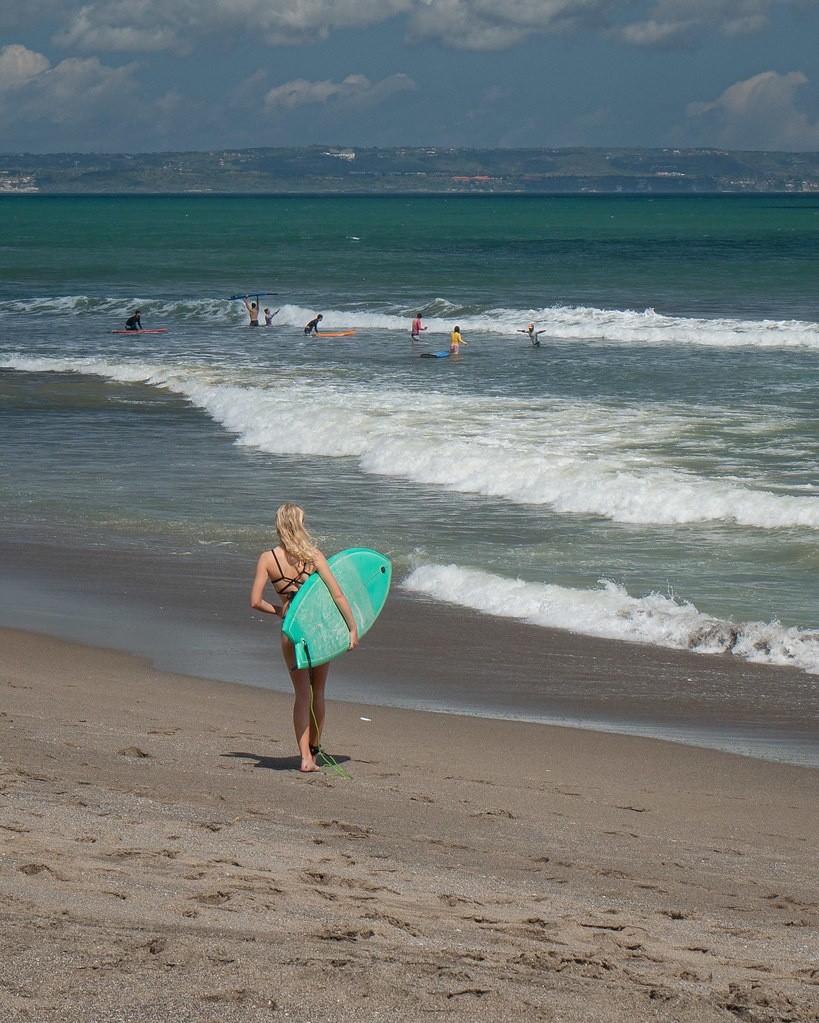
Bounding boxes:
279,547,392,669
110,327,170,335
229,293,277,300
313,329,358,339
421,350,448,360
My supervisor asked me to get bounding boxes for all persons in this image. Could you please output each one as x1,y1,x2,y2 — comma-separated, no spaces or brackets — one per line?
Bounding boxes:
264,307,281,325
410,312,427,341
517,323,546,345
125,311,142,332
251,503,359,773
245,293,259,326
450,326,467,354
304,313,323,336
